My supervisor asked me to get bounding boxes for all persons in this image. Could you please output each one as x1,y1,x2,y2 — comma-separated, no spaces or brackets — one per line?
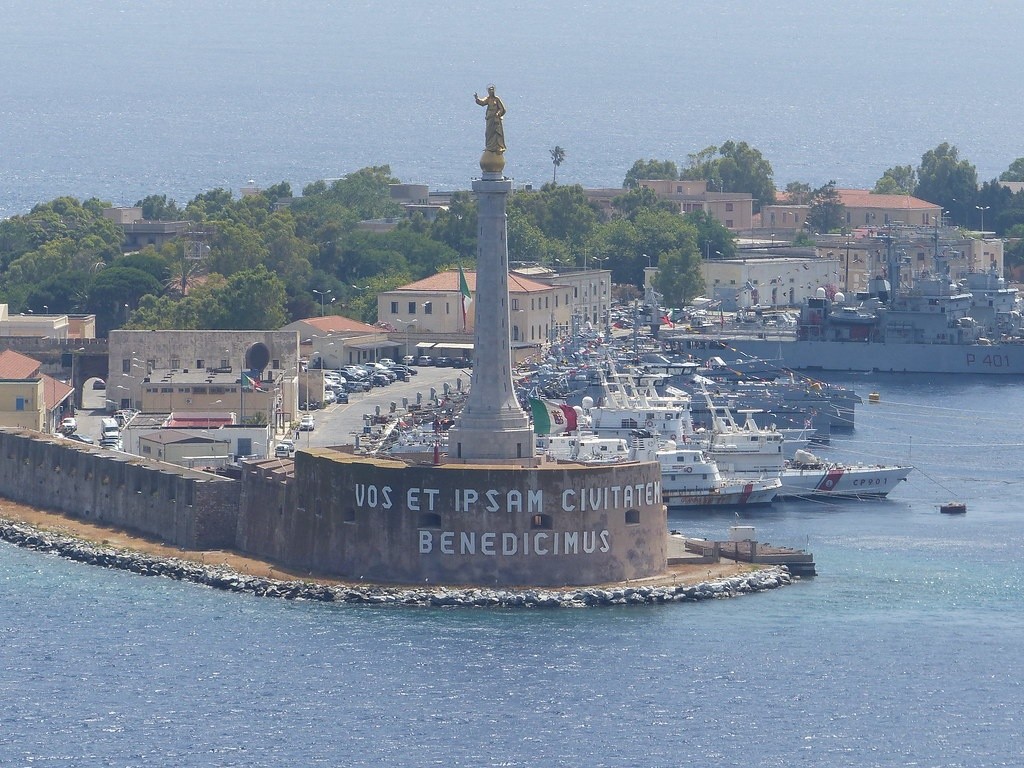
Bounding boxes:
472,84,505,154
295,426,300,439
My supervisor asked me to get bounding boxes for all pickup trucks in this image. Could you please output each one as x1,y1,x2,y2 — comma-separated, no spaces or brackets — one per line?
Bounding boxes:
674,305,708,316
750,304,771,312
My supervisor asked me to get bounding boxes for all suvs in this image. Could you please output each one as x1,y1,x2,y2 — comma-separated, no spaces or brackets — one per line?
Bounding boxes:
379,358,396,367
301,415,315,431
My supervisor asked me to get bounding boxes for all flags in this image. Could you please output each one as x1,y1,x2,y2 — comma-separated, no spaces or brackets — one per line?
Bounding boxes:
528,395,578,434
457,267,474,329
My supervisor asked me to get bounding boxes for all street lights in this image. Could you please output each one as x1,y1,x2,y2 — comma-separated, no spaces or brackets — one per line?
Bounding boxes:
593,257,609,268
207,400,222,434
312,334,332,369
116,386,131,420
976,206,990,231
43,306,48,313
352,285,369,321
72,348,85,389
202,430,216,476
313,290,332,317
716,250,724,260
556,259,570,268
943,211,949,229
132,358,146,379
306,351,320,448
125,304,128,325
320,343,334,369
644,255,651,267
253,442,268,459
397,318,417,376
106,399,119,450
122,373,136,417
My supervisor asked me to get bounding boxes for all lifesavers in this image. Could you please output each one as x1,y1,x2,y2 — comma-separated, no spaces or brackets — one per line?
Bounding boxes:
687,467,693,473
647,421,653,427
671,434,676,440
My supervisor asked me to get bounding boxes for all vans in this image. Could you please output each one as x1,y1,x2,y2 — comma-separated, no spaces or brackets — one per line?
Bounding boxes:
101,418,122,440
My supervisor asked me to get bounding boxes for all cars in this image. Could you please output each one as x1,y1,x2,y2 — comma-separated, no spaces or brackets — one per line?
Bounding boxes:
96,439,122,450
452,357,469,368
62,417,77,429
67,434,93,444
468,360,473,369
618,311,628,317
610,314,620,322
275,445,290,458
280,438,296,453
402,355,417,365
418,355,435,366
94,379,105,389
686,325,701,334
321,363,418,403
435,357,454,367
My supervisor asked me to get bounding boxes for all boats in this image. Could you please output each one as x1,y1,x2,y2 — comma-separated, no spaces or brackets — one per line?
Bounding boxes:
387,297,913,507
659,213,1024,373
